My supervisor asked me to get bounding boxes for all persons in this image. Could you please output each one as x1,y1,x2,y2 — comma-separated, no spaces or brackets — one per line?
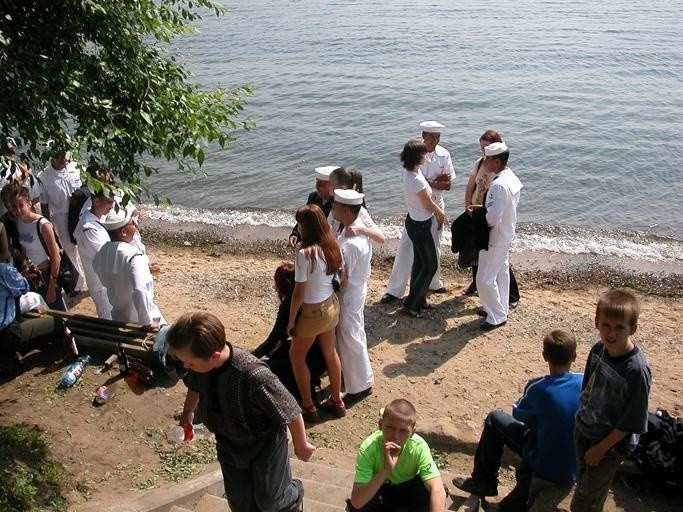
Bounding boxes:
286,204,346,423
74,189,116,319
326,167,386,245
289,166,342,249
0,262,68,369
0,184,23,274
331,189,374,406
92,205,167,332
0,158,41,244
344,399,453,512
250,263,327,408
570,289,652,512
467,142,524,332
400,140,446,319
78,163,147,255
165,311,316,512
68,155,100,240
452,328,584,512
37,139,89,298
465,130,520,308
381,121,456,304
8,187,69,312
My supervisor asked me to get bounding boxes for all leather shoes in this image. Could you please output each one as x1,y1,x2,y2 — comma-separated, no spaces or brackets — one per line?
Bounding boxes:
433,287,448,294
476,309,487,319
479,322,506,331
463,284,476,296
510,300,518,310
381,292,395,303
343,386,374,405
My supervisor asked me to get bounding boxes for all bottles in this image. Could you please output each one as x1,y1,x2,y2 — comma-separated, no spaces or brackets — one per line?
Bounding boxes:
161,423,206,443
59,355,91,385
96,386,107,404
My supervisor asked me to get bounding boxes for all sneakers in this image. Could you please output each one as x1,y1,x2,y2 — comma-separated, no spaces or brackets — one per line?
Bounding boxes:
452,475,499,496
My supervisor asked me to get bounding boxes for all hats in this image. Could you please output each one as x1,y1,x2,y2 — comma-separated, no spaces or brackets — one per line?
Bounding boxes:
331,189,364,205
315,166,341,181
483,141,508,156
44,134,72,150
96,205,136,231
420,121,443,133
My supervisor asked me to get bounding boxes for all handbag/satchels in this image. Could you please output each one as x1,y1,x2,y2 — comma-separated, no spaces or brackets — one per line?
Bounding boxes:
37,217,79,292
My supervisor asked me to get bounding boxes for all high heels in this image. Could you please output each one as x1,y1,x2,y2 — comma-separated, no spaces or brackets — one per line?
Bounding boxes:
297,404,321,425
320,397,347,418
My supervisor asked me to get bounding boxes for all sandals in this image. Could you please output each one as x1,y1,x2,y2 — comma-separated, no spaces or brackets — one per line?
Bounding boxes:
421,302,435,309
405,307,424,318
